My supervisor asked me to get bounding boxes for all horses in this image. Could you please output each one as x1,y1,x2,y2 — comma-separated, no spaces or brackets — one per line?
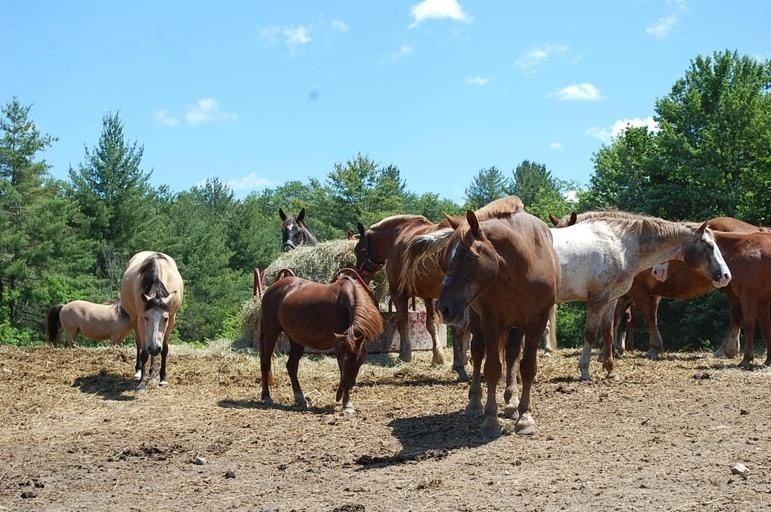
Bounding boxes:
354,212,472,373
120,251,185,394
259,273,386,418
47,298,132,351
277,207,320,256
549,193,771,400
433,193,563,437
